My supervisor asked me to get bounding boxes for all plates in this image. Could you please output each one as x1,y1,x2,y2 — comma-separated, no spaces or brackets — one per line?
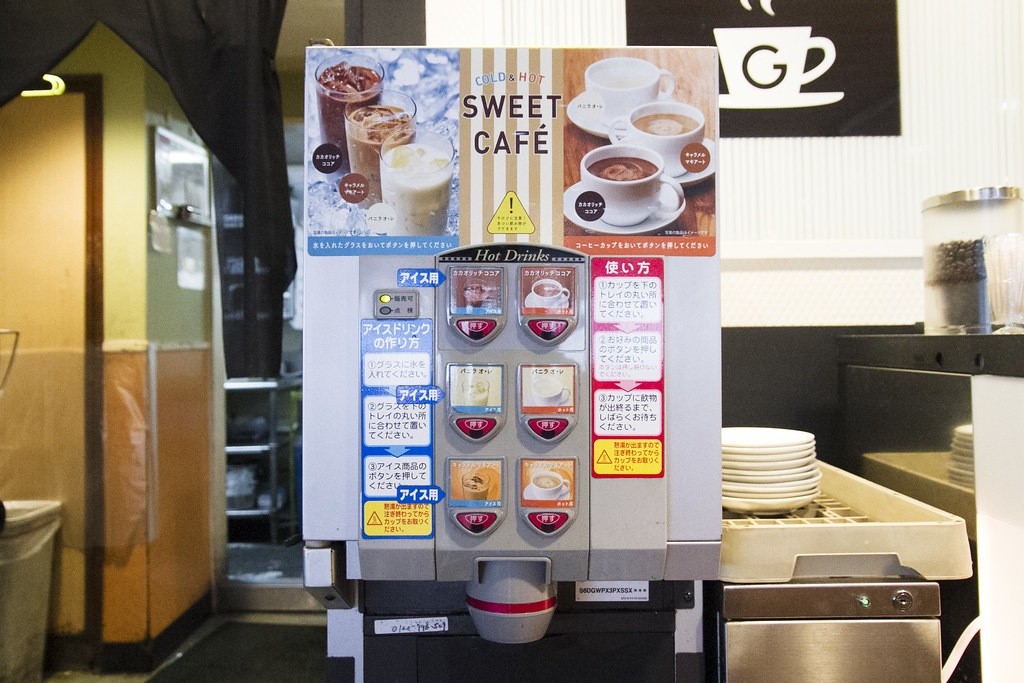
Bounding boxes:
523,483,570,500
566,90,676,139
563,181,686,236
609,118,717,186
525,292,569,308
947,425,974,489
722,427,824,516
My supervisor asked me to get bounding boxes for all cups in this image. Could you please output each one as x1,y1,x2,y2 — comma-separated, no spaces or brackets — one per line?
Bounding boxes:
628,101,705,178
531,279,570,308
580,144,685,227
461,378,490,406
344,89,417,201
462,278,490,307
584,57,676,131
530,470,571,500
380,127,455,236
315,54,385,171
462,472,490,500
530,379,570,406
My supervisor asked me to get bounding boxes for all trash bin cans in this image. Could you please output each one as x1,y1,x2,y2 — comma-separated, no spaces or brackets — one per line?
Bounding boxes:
0,499,66,683
